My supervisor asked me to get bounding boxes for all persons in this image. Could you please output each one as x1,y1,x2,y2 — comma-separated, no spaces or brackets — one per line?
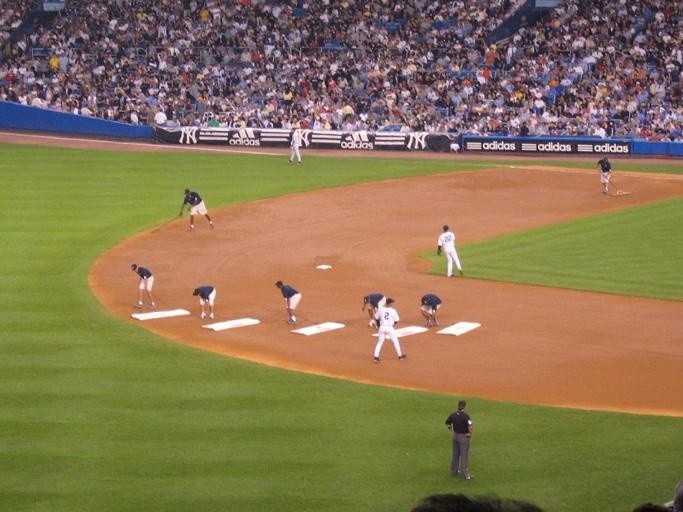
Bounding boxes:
178,187,213,230
287,125,302,165
192,285,216,319
417,293,441,327
595,155,611,194
435,224,462,279
273,279,301,324
446,398,475,481
130,263,156,309
361,292,386,326
0,1,682,142
372,297,406,363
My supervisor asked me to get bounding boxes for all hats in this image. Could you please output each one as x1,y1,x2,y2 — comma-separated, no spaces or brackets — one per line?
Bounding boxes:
422,297,430,305
363,296,371,305
386,298,395,304
130,264,137,270
193,288,199,296
457,400,466,410
274,280,282,286
184,188,189,195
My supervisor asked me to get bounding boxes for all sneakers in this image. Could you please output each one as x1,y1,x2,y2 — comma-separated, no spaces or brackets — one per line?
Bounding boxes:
187,220,214,231
132,302,157,309
373,355,406,363
287,318,297,324
367,320,439,328
451,470,475,480
199,313,213,320
